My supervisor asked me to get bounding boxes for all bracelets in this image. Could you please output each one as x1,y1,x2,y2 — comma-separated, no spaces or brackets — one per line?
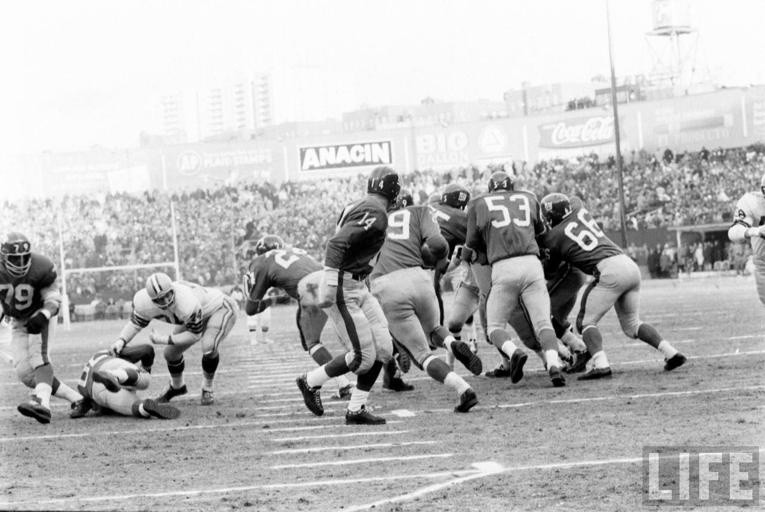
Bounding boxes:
118,336,127,348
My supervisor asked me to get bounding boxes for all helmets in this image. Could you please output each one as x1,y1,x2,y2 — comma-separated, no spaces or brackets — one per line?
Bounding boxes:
147,273,176,314
255,236,283,254
0,233,32,278
367,167,571,228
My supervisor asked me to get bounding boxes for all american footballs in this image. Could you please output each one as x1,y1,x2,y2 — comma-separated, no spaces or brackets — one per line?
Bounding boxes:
422,241,437,266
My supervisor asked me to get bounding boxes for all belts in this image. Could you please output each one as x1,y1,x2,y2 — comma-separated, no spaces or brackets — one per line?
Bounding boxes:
352,273,365,281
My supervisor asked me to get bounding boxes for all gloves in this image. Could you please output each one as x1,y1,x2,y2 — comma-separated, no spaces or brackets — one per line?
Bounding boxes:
151,328,172,347
109,339,125,359
26,311,50,333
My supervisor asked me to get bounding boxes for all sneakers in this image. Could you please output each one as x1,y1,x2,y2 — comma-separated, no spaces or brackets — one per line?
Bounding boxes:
18,403,51,424
91,371,121,392
143,384,215,420
70,397,91,417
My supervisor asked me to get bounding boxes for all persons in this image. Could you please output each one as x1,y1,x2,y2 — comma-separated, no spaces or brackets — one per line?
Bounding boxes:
0,147,765,321
360,162,688,416
240,231,412,400
76,341,180,423
294,165,403,426
110,270,241,408
0,230,94,428
